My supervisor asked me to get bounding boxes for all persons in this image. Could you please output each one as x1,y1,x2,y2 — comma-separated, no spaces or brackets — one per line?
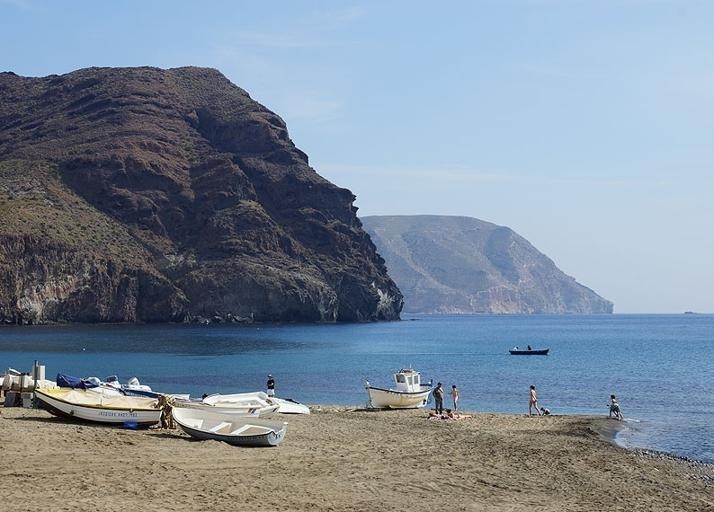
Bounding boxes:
429,412,450,419
528,385,541,417
528,345,531,350
450,385,459,410
266,374,275,397
612,399,623,420
541,408,550,416
433,382,444,414
447,409,454,418
154,394,177,429
609,395,617,417
514,346,518,351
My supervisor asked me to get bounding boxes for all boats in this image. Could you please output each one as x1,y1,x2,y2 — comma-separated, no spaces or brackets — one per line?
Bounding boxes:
365,369,434,409
509,348,549,355
